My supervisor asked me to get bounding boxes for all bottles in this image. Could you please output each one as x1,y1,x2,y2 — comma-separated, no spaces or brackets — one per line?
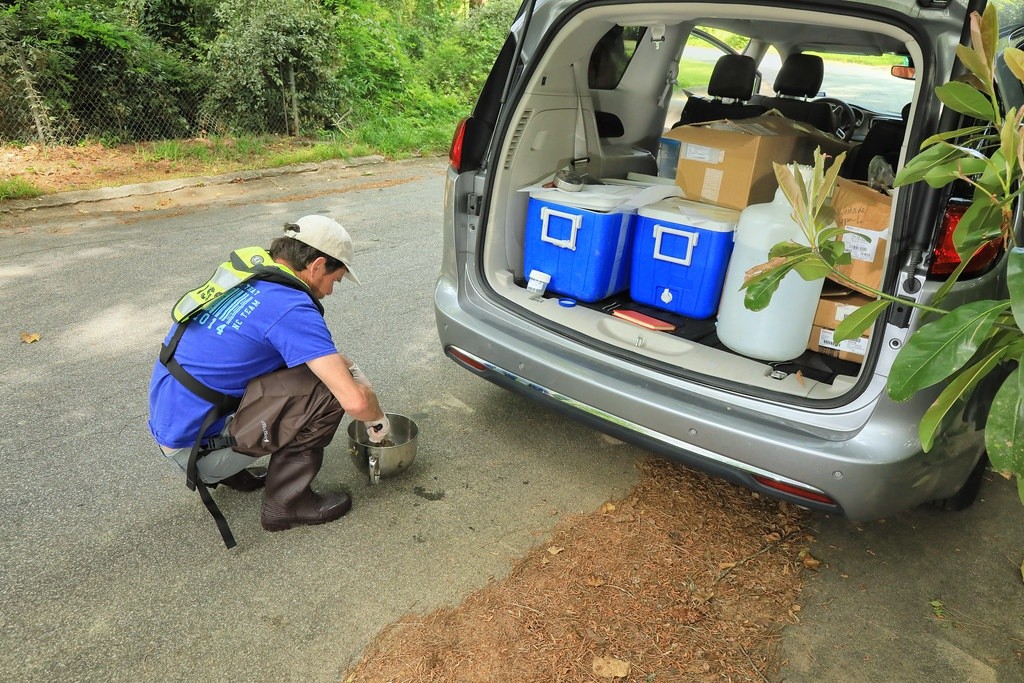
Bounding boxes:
715,165,838,363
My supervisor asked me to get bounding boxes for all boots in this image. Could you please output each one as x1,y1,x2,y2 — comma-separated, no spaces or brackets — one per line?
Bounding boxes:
259,446,351,533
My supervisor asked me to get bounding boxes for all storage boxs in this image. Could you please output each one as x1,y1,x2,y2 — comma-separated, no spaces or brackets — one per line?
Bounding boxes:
807,289,875,364
822,176,893,298
660,115,850,212
630,197,742,319
523,182,654,303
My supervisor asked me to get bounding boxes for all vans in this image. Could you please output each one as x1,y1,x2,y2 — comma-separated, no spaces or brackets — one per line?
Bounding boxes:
431,0,1024,529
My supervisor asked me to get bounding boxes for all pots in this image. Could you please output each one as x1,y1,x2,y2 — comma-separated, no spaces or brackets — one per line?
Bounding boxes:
347,413,419,486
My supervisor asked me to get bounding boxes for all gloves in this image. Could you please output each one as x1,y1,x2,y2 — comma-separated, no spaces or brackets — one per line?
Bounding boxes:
349,363,371,387
364,409,390,443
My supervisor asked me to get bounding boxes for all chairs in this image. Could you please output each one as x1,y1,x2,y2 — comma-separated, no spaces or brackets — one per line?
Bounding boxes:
748,52,836,136
672,54,767,129
854,102,912,180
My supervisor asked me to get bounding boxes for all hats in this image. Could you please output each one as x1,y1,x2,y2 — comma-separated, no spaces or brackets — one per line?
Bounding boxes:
286,214,362,288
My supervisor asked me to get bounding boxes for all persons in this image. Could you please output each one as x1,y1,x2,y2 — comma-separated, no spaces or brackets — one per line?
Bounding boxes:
149,215,391,532
